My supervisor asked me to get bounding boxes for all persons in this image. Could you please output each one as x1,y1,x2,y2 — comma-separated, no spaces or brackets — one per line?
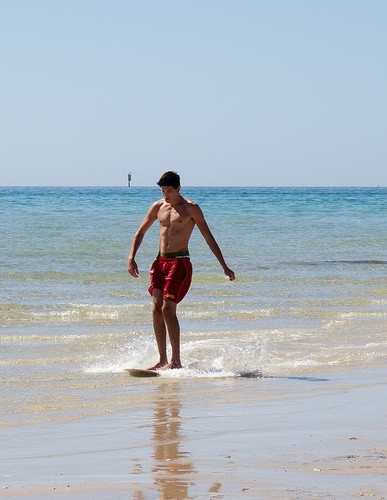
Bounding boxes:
127,170,237,371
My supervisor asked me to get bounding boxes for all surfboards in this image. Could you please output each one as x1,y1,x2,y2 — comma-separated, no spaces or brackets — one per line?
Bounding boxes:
124,367,159,378
